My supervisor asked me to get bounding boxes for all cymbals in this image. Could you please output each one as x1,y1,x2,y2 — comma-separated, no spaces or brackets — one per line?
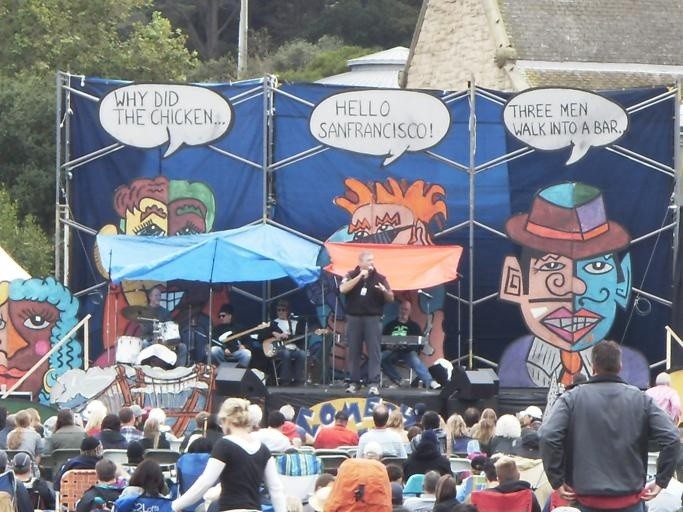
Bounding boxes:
119,304,155,324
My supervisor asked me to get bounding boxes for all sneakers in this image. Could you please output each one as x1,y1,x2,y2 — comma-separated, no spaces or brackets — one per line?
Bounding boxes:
345,384,358,393
367,387,380,396
388,377,406,389
429,381,442,390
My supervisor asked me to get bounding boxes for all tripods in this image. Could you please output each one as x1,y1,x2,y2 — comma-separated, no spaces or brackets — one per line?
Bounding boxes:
290,322,329,392
326,296,345,389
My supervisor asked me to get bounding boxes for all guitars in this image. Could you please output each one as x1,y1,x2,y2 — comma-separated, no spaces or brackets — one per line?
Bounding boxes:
263,328,329,358
197,321,271,363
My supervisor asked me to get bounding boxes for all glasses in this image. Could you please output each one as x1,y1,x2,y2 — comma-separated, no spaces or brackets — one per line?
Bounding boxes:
217,314,231,319
277,308,287,312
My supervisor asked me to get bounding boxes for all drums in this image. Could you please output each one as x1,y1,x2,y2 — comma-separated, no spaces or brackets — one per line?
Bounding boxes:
115,335,142,364
155,320,181,343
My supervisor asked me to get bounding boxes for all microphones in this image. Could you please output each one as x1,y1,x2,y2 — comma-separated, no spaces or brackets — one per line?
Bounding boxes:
361,265,373,277
417,289,432,299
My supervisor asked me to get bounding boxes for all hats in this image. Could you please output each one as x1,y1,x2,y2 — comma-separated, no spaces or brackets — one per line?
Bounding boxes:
411,402,427,417
519,405,543,420
79,436,101,453
128,404,148,418
503,179,633,262
11,451,32,468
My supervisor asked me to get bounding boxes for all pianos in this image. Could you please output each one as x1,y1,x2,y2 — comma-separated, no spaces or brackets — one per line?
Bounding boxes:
379,334,428,348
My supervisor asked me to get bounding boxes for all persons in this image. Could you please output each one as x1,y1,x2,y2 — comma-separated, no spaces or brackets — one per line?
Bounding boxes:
2,373,581,512
381,301,442,390
643,372,683,512
260,301,310,389
540,340,681,512
339,252,394,396
211,304,251,368
121,288,188,367
